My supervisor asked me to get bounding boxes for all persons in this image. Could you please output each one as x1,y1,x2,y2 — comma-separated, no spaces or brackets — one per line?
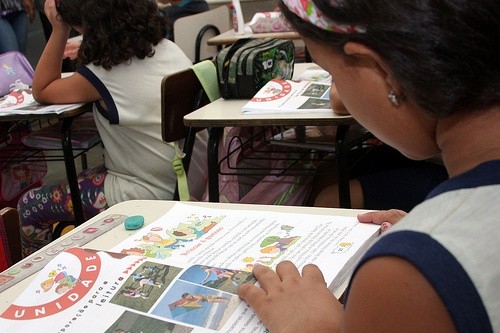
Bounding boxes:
173,291,231,311
0,0,35,56
197,265,240,288
159,0,211,43
311,77,451,217
237,0,500,333
120,266,165,301
16,0,212,261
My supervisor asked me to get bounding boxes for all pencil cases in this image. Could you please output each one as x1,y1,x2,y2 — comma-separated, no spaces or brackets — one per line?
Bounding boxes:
0,50,34,97
249,11,294,33
216,37,296,99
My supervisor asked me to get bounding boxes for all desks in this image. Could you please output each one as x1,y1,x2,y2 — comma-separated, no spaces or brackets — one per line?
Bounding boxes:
0,199,389,333
183,62,354,209
208,20,312,62
0,71,102,227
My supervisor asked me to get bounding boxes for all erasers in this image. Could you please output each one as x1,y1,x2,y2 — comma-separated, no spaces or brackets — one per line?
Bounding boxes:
125,215,144,230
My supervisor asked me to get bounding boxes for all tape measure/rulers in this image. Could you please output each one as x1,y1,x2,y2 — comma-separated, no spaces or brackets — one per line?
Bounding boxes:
0,214,129,292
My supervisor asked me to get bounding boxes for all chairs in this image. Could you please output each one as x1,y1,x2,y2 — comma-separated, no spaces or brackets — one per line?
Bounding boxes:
0,208,22,269
160,61,220,201
173,5,230,64
